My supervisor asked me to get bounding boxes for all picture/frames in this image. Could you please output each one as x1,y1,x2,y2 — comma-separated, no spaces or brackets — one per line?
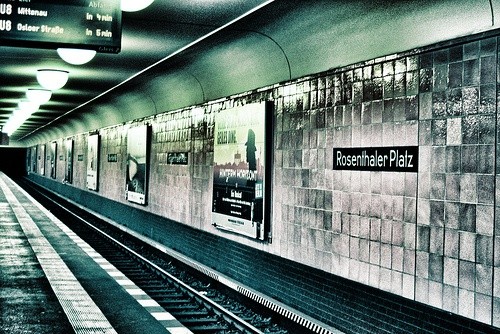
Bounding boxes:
85,133,99,190
64,140,73,184
126,124,148,205
26,141,57,179
210,100,272,240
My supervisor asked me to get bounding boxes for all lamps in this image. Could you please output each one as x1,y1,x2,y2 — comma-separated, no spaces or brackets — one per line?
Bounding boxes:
27,88,52,106
56,46,96,66
18,100,39,113
118,1,155,13
36,68,69,89
